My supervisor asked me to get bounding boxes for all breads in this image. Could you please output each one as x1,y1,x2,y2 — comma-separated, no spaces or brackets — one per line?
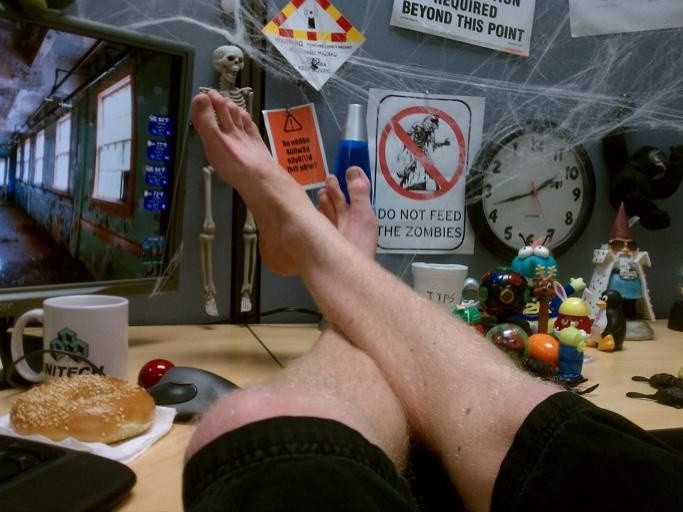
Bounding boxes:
8,375,157,445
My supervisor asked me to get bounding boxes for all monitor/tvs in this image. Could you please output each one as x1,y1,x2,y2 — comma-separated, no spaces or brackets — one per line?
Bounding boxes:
0,2,195,318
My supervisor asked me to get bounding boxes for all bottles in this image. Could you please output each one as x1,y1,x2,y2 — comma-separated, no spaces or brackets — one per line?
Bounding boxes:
333,103,373,202
142,237,149,257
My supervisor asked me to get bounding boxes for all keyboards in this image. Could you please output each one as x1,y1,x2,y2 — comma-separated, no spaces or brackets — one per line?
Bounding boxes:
0,420,136,512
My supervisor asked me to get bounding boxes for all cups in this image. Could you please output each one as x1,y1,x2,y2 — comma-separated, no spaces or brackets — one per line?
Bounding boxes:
410,262,468,312
9,295,129,384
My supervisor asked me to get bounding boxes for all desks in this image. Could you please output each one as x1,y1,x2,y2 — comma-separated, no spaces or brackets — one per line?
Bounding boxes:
1,319,682,512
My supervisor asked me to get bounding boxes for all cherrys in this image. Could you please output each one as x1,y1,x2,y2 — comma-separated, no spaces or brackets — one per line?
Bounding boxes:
137,360,174,387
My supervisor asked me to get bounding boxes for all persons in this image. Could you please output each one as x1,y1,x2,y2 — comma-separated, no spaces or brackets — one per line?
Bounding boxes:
453,200,656,394
201,47,257,318
182,89,683,512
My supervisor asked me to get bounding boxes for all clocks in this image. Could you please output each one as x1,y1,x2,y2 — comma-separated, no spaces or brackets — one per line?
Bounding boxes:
466,120,595,262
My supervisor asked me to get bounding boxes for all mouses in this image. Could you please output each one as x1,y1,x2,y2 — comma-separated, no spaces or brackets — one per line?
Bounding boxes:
136,358,242,418
